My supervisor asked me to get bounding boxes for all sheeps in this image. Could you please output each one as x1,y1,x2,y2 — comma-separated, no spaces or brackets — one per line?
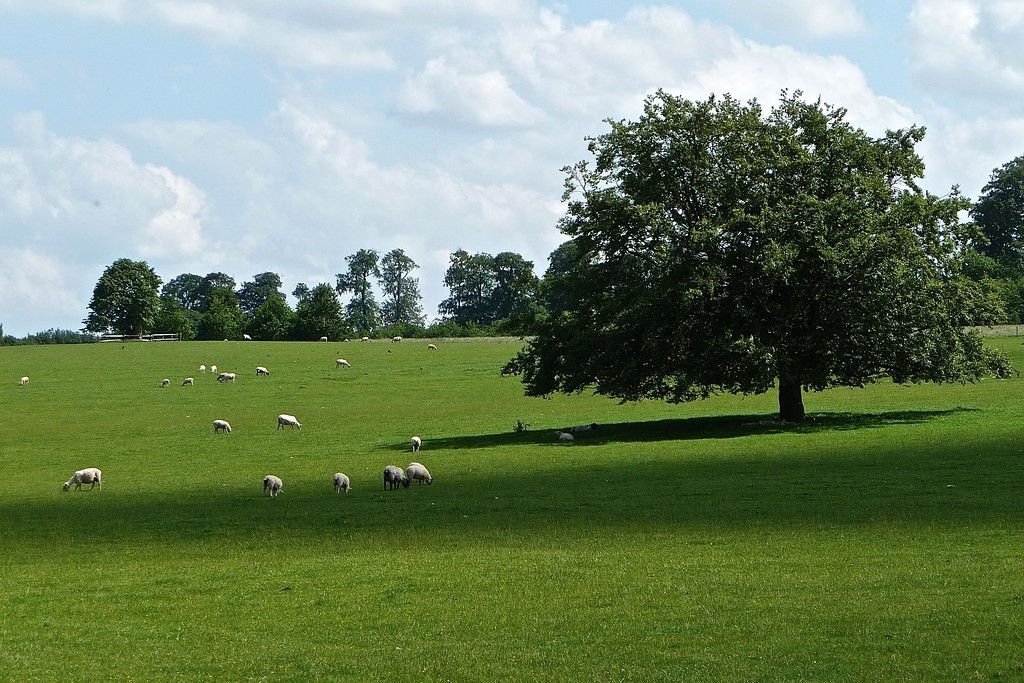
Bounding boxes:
213,419,231,434
344,339,349,342
411,436,421,451
243,334,251,341
210,365,217,374
391,336,402,343
256,367,269,376
62,468,103,492
199,365,205,374
278,414,302,430
361,337,368,342
20,376,29,385
554,423,598,441
263,475,283,497
333,473,352,493
216,372,235,383
336,359,350,368
224,339,228,342
182,378,193,386
320,337,327,342
383,462,435,491
160,379,170,387
428,344,437,350
